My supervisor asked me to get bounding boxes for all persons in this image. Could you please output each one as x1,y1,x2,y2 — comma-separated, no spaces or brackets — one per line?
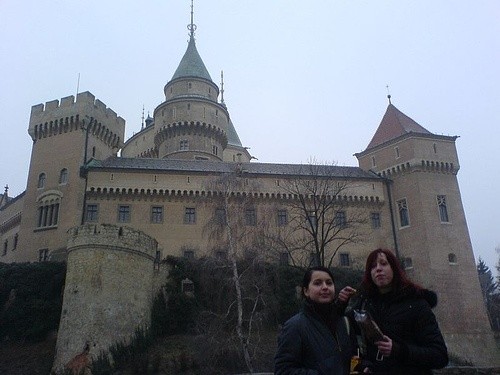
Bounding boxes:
334,248,449,375
272,266,352,375
